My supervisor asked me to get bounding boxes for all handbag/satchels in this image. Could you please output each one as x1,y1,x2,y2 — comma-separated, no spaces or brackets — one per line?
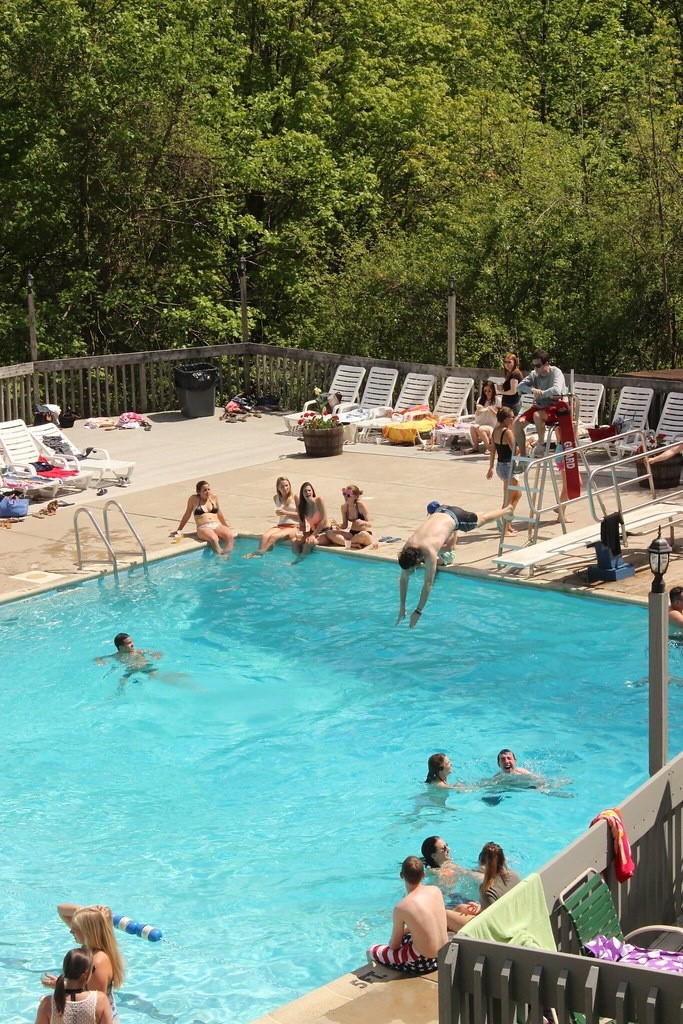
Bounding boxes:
0,494,29,517
586,424,617,443
320,391,343,414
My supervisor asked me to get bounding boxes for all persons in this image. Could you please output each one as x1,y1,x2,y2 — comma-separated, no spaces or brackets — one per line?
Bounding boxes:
242,477,373,567
490,749,573,800
35,903,125,1024
95,633,164,692
170,480,234,561
668,586,683,627
364,836,522,974
396,349,582,629
644,443,683,466
412,753,471,817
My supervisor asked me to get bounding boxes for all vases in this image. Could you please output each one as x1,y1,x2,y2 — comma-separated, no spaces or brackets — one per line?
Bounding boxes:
302,424,346,457
636,454,682,489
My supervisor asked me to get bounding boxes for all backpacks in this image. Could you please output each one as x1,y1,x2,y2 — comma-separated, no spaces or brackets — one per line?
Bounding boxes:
31,404,81,428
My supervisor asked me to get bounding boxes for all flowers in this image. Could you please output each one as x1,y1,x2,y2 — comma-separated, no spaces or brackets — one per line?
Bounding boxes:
636,431,669,459
298,385,340,429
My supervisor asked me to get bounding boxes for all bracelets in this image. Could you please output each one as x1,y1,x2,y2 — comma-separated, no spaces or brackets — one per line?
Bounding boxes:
415,609,421,616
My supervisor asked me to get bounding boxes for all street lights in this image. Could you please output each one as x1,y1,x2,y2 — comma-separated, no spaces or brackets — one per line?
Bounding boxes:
447,273,456,365
239,256,248,341
24,270,36,359
646,525,671,776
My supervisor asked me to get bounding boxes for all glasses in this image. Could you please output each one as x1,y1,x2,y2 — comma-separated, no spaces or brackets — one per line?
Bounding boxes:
91,965,96,974
342,489,355,498
68,928,83,937
504,361,513,364
508,415,515,420
440,844,448,852
530,363,543,369
483,390,492,393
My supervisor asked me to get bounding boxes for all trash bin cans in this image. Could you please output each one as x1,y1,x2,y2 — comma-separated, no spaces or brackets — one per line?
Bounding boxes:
173,362,219,418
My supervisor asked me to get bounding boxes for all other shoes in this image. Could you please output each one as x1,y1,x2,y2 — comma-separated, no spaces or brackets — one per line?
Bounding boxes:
0,517,25,529
391,440,441,452
534,443,544,457
343,439,355,445
518,462,527,471
449,445,465,452
378,536,401,542
219,412,262,423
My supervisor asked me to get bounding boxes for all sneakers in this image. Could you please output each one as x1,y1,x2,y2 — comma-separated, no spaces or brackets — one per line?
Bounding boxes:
117,476,134,487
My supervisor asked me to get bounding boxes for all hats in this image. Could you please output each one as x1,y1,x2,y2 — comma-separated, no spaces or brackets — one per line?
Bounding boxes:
428,502,440,513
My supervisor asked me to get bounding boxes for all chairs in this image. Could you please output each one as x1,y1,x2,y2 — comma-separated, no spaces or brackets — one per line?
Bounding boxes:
1,418,136,500
558,868,683,1024
283,365,683,463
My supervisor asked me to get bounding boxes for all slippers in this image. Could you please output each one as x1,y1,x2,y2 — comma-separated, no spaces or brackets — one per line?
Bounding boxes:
32,499,75,519
468,449,480,454
140,422,152,431
96,488,108,495
485,450,491,455
